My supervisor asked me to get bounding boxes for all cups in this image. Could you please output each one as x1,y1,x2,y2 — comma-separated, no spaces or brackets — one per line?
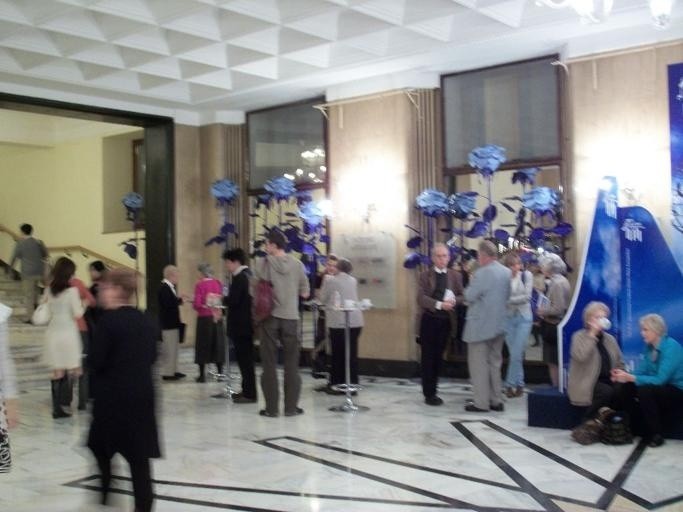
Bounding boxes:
599,317,611,330
329,292,371,308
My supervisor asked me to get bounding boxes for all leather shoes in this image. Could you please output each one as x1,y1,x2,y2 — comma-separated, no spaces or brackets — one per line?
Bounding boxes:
465,403,504,411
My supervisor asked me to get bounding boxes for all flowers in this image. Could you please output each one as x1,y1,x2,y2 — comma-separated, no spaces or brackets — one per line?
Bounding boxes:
197,172,334,293
118,188,148,308
400,144,576,322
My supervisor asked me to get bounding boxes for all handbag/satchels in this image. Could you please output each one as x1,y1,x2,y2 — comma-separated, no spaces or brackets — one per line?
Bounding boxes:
251,281,272,322
531,289,538,315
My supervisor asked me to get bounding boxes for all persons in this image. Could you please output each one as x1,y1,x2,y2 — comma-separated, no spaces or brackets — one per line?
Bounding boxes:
30,257,107,417
160,265,186,381
532,252,572,388
212,247,258,402
610,313,683,447
249,226,310,417
1,304,20,474
193,263,224,383
313,255,363,394
416,239,534,412
84,268,166,511
8,223,49,325
567,301,627,423
